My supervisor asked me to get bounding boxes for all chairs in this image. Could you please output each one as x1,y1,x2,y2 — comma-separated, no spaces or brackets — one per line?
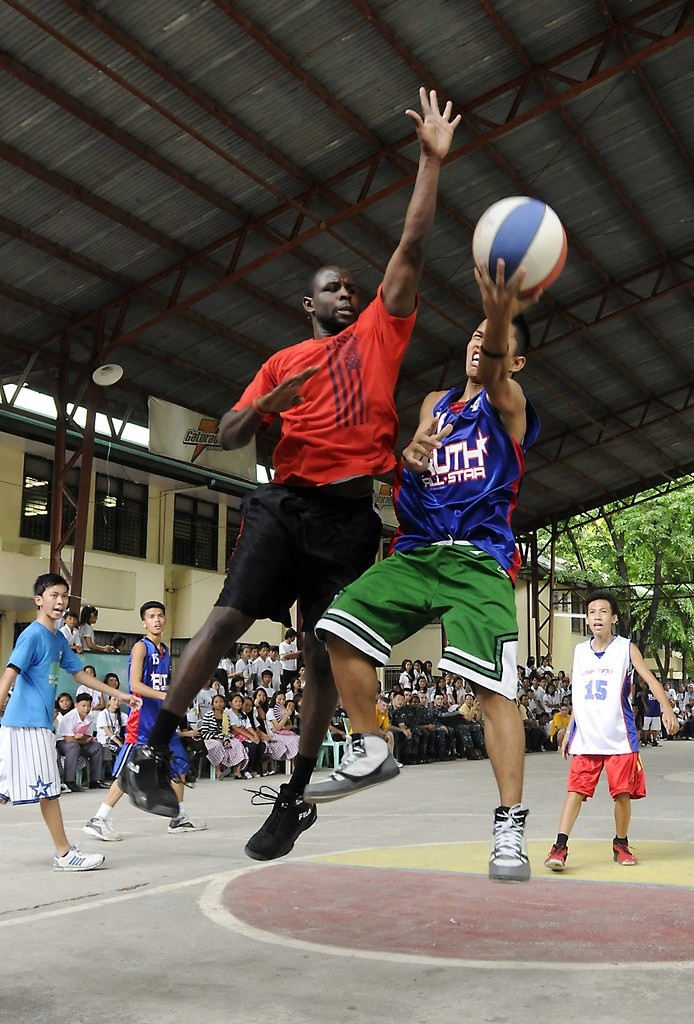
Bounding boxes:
52,718,350,788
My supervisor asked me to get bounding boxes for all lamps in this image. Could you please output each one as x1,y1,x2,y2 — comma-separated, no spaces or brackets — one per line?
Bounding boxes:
88,494,127,510
90,359,124,387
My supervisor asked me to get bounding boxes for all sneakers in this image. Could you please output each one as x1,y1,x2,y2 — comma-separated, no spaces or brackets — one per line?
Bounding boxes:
244,782,319,861
83,816,122,842
53,844,106,872
167,814,208,834
116,744,188,822
302,729,401,806
612,838,638,865
545,843,569,872
60,783,72,793
488,802,531,882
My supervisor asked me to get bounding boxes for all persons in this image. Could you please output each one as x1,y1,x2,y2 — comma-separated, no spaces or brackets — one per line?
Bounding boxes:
304,258,541,882
632,672,694,747
0,573,143,872
177,628,349,782
0,693,11,717
545,593,679,869
54,606,127,653
54,665,128,794
349,659,489,767
82,601,207,840
117,86,462,862
517,655,572,753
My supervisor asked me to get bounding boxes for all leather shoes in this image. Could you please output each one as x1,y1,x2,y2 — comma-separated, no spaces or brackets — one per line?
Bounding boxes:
66,782,84,792
88,781,111,789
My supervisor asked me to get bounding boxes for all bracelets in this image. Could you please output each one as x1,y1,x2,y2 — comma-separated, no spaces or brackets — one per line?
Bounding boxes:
253,395,270,416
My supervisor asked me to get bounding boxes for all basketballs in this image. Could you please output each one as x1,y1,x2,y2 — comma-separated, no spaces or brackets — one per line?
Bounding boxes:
472,195,569,301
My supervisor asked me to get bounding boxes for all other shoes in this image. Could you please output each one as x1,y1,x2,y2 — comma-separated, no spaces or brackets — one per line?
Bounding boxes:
395,746,490,768
251,771,260,778
651,742,663,747
232,774,247,780
185,773,197,783
244,772,253,779
217,767,231,781
262,770,275,778
640,741,647,747
